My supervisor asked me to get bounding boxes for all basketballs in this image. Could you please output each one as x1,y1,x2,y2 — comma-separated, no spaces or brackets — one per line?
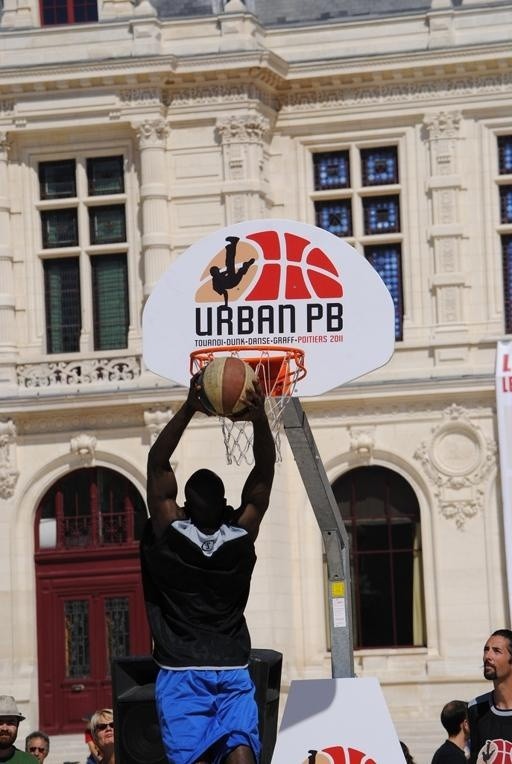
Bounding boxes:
198,357,255,416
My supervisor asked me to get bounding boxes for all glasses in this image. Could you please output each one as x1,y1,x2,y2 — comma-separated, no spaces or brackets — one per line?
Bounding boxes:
28,747,45,753
92,721,113,732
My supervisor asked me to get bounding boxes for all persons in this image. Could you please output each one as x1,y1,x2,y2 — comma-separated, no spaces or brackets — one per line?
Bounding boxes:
88,707,115,764
141,366,276,763
23,731,51,764
85,721,104,763
429,699,470,762
467,628,512,763
400,742,415,764
0,694,42,763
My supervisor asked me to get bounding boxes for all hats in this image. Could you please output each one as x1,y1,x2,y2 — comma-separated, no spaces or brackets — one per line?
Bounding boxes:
0,694,27,723
